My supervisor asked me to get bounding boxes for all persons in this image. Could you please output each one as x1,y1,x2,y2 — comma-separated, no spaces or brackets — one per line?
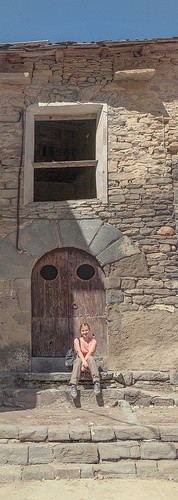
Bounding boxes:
71,323,101,398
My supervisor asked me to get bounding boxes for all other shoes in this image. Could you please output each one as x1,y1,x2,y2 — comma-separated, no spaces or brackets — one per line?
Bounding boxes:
71,384,77,399
94,381,101,396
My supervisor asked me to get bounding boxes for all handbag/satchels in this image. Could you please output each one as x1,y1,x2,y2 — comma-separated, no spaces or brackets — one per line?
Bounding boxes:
65,336,80,367
